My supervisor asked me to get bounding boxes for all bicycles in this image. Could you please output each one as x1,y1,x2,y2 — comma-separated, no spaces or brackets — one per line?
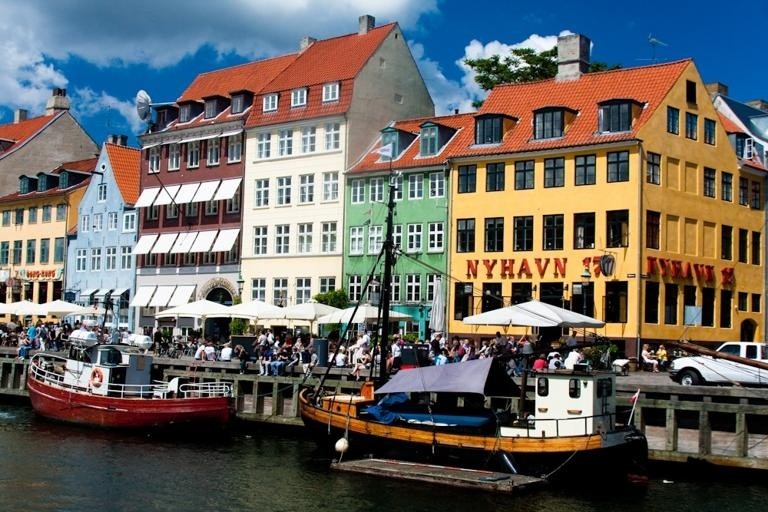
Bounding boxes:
157,340,185,360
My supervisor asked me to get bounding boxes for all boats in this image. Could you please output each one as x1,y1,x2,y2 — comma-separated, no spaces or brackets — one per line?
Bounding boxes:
285,151,661,492
26,330,237,438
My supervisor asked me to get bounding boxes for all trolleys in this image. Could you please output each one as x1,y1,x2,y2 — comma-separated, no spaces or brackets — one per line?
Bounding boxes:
612,357,630,376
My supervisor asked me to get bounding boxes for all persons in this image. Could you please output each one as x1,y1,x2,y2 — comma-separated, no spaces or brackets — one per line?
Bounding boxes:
642,344,667,372
388,336,428,370
1,320,81,360
154,326,247,375
258,329,317,377
328,330,381,380
429,331,591,372
93,326,133,346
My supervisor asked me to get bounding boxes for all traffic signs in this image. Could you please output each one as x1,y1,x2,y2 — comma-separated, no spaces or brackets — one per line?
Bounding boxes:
626,273,636,279
640,273,651,279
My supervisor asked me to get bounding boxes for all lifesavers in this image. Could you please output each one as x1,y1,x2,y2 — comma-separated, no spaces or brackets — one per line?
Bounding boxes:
91,368,103,387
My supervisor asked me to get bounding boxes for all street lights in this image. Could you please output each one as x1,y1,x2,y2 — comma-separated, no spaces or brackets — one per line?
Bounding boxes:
577,260,593,349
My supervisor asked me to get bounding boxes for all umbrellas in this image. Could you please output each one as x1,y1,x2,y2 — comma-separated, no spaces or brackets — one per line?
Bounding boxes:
317,303,415,334
226,298,283,333
7,299,39,326
39,298,84,326
283,301,344,335
65,303,114,319
154,297,226,340
463,300,609,344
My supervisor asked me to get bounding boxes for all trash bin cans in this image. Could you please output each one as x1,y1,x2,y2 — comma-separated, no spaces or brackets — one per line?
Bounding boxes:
399,343,429,370
313,339,328,367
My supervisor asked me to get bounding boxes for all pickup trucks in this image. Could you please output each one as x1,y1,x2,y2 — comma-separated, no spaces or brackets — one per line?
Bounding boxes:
665,340,768,389
